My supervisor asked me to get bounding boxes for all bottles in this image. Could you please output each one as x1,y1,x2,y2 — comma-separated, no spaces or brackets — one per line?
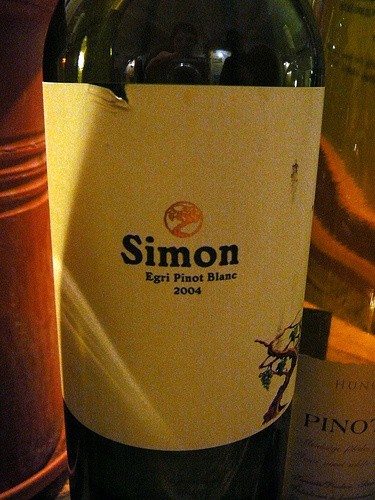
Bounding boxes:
41,0,328,500
282,0,374,500
